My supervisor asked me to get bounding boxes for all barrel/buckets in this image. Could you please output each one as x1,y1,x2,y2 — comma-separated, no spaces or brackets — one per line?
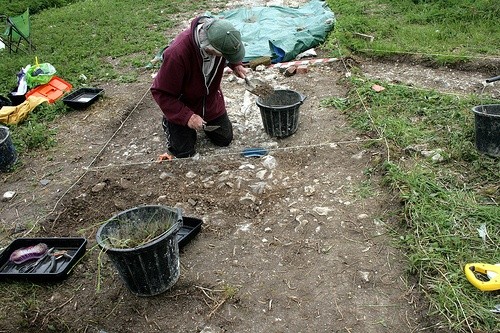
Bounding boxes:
0,126,18,172
95,205,183,297
255,89,307,138
471,104,500,158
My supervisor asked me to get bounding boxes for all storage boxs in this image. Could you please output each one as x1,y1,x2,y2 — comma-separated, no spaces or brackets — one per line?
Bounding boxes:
177,216,203,248
0,237,88,285
62,87,104,110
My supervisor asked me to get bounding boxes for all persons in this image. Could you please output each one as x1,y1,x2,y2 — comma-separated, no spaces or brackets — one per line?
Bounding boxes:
150,16,247,158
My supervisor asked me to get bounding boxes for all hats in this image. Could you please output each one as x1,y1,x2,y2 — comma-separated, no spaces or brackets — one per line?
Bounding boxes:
207,20,247,61
